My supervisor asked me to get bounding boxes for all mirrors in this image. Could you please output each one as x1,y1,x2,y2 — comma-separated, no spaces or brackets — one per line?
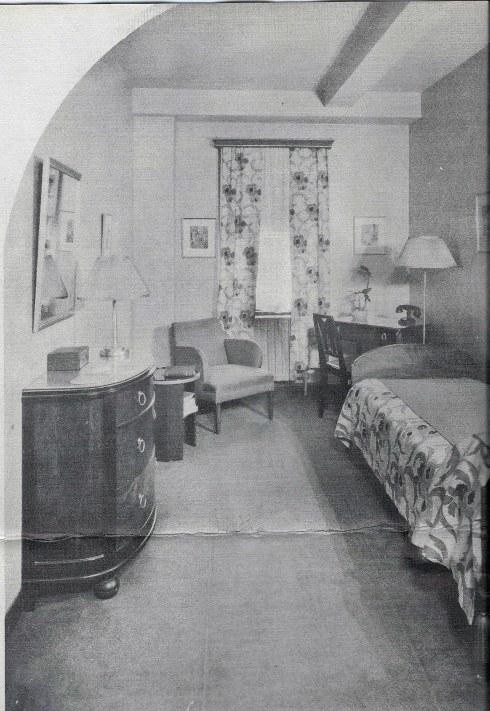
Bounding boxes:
33,158,83,334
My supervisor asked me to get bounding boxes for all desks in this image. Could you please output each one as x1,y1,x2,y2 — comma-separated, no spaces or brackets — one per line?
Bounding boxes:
334,314,430,358
153,365,201,463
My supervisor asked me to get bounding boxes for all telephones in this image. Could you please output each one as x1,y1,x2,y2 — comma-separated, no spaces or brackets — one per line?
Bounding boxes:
396,305,421,326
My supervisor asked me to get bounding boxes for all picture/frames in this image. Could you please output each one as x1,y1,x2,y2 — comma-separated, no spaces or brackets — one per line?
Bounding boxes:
353,215,388,256
181,218,217,259
475,191,490,254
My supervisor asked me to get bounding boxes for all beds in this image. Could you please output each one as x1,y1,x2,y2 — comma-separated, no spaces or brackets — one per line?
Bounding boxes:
351,342,489,572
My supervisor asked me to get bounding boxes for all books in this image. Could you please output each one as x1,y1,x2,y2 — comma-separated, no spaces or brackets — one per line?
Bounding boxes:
184,392,198,418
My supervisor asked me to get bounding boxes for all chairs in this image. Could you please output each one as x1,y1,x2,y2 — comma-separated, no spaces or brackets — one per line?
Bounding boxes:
313,312,360,420
171,318,275,434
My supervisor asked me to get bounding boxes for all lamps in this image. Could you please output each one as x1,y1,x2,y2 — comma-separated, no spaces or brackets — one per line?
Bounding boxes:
78,255,152,359
394,236,460,345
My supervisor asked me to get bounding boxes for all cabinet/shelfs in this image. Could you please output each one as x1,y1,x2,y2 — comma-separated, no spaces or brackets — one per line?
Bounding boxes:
20,355,159,613
